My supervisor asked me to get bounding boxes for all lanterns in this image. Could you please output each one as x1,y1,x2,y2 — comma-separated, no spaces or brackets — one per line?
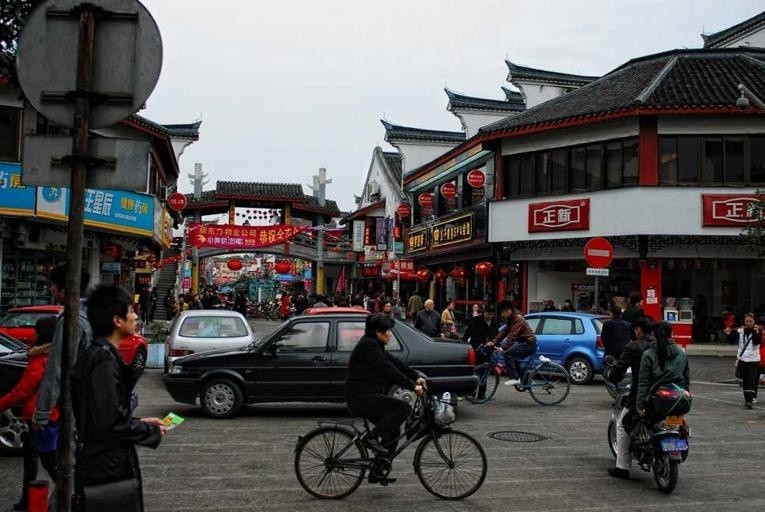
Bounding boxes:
434,268,448,282
450,267,468,281
475,261,494,275
275,262,290,275
226,259,242,270
416,268,433,282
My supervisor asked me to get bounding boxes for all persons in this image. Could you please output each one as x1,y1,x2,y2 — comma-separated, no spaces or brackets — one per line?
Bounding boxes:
32,262,94,511
0,316,64,511
71,283,168,512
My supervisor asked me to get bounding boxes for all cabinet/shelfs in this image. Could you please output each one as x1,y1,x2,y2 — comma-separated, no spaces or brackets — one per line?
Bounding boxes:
0,253,54,306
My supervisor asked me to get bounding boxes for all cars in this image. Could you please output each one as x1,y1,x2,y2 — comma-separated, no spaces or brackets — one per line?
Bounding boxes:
489,311,612,386
1,305,148,379
160,310,257,374
160,314,481,419
304,307,372,344
0,330,139,454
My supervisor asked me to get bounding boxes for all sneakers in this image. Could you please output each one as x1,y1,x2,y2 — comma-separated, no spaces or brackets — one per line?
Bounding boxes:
606,467,631,480
503,378,521,386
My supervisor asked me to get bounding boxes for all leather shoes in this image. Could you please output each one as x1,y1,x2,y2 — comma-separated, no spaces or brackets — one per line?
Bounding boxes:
367,470,398,484
361,436,390,455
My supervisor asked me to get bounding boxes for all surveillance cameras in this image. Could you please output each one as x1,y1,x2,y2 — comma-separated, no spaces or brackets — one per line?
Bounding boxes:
736,96,749,111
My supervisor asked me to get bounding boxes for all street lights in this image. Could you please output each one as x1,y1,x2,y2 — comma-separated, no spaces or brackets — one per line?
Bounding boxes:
388,251,401,306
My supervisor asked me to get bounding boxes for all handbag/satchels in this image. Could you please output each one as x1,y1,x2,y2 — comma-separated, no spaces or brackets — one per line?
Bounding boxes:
735,359,743,380
79,476,141,512
405,389,447,442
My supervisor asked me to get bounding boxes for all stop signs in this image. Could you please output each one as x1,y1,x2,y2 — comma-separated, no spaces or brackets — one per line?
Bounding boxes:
584,238,614,267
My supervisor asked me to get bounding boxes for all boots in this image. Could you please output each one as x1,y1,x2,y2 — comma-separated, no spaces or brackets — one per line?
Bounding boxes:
743,389,755,409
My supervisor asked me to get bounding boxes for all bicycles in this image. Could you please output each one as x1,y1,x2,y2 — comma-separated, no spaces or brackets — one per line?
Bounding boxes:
465,344,572,407
293,382,488,501
256,298,281,320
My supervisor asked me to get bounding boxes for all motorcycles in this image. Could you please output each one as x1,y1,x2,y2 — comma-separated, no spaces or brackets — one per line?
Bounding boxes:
602,352,692,495
